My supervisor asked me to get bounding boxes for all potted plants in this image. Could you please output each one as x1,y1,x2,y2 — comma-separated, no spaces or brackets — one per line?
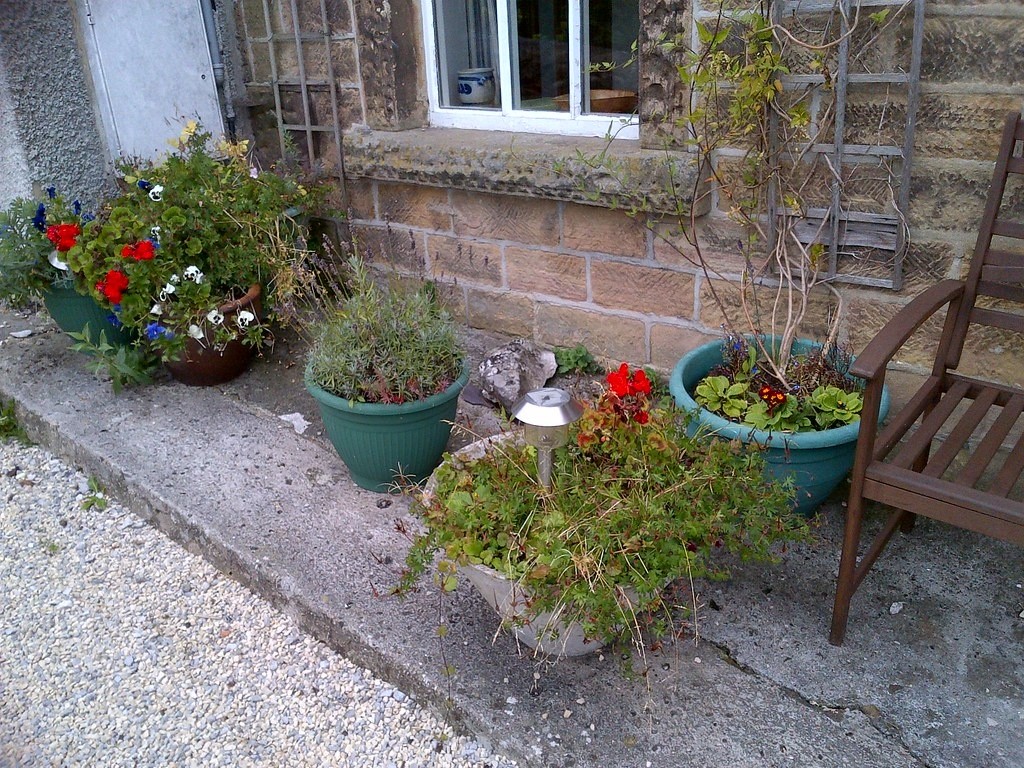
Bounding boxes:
554,3,888,529
267,208,474,492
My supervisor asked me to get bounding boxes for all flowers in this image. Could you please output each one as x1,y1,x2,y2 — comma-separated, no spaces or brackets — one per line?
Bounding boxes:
1,103,307,395
370,357,825,707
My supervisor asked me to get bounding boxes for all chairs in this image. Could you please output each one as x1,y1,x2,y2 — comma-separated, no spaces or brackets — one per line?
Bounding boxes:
828,106,1024,646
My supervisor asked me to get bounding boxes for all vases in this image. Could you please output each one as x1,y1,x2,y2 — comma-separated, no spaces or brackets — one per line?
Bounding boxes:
40,201,310,387
421,430,681,657
458,67,495,107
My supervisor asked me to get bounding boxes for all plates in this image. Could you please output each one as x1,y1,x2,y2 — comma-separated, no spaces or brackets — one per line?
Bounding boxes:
553,89,636,112
